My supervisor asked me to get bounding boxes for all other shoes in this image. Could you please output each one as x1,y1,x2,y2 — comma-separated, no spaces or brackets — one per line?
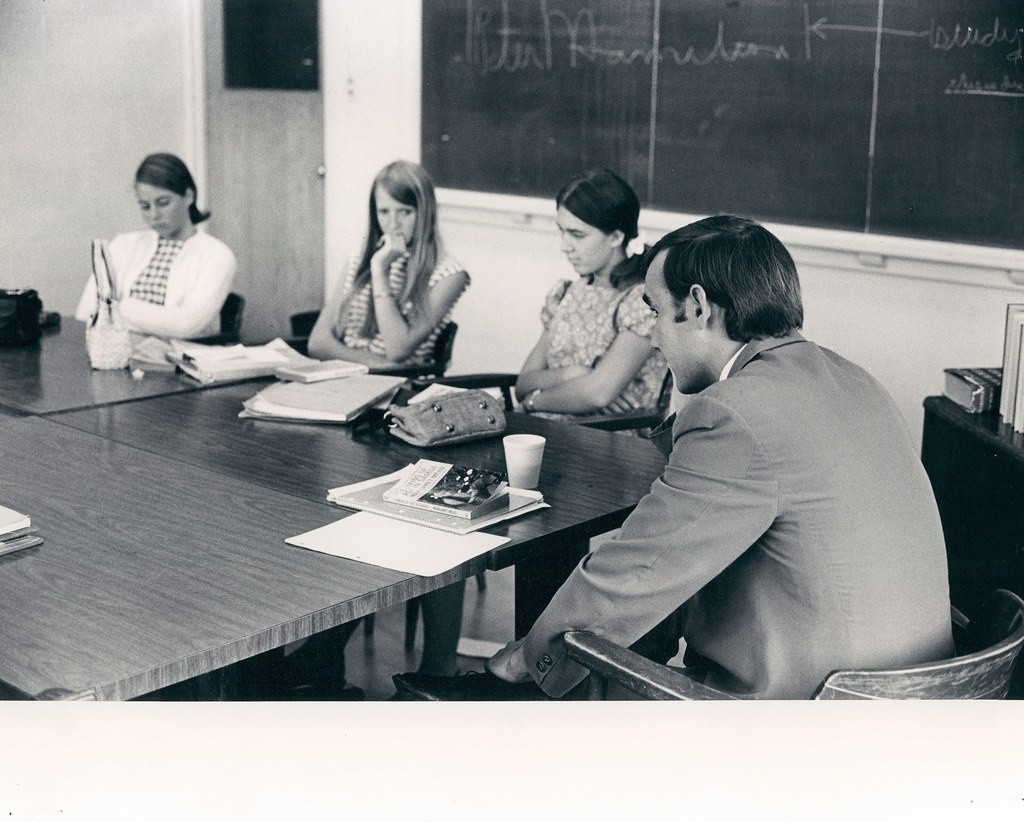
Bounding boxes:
275,652,346,693
238,646,285,682
392,670,549,701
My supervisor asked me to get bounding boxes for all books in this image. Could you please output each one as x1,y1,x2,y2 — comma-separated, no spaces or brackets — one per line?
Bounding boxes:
943,304,1024,433
0,507,43,554
129,335,407,423
387,459,510,519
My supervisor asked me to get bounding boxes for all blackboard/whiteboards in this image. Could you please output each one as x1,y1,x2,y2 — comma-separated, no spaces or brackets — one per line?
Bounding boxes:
418,0,1024,275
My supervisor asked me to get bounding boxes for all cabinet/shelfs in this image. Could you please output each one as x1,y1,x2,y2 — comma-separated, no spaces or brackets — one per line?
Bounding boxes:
922,398,1024,700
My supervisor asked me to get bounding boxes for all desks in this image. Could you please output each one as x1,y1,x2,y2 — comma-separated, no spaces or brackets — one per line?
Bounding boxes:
39,375,668,644
0,316,276,418
0,401,490,702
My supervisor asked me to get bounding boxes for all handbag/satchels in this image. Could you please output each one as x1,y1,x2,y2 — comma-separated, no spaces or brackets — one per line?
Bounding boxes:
0,288,43,352
86,237,132,372
351,389,507,447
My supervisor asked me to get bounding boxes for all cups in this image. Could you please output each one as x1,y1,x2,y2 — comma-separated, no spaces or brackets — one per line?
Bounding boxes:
503,434,546,489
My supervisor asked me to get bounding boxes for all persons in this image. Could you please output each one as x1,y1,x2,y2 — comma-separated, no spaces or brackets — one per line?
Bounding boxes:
223,167,674,701
74,154,236,339
307,160,471,369
393,215,956,700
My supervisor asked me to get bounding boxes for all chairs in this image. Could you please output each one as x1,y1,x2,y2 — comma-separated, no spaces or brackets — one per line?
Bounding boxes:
281,309,457,381
413,365,673,437
219,292,245,341
558,588,1024,701
